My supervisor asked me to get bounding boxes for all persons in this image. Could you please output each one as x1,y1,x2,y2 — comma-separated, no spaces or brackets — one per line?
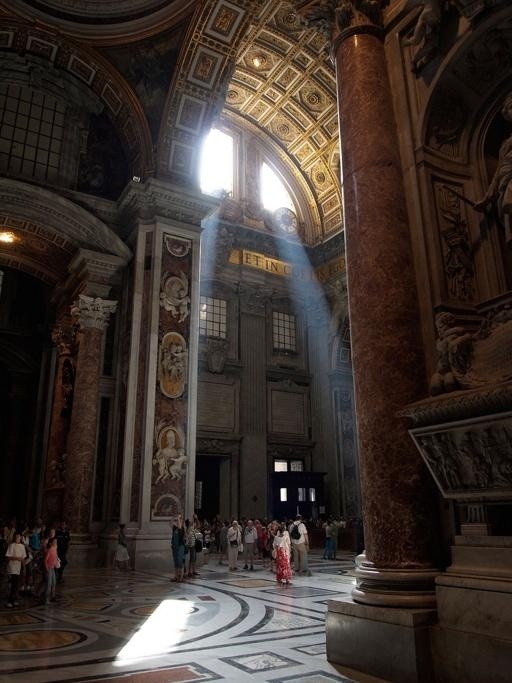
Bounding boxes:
0,518,71,608
159,292,181,319
154,430,191,485
176,289,191,323
162,344,185,382
115,523,133,570
171,511,347,584
419,426,512,491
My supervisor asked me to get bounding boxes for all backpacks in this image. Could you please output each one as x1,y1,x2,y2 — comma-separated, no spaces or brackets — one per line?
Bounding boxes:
290,521,304,540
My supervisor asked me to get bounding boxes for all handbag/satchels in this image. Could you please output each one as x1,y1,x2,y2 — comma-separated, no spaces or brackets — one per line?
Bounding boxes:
195,538,203,552
53,557,62,569
271,549,278,559
230,539,238,546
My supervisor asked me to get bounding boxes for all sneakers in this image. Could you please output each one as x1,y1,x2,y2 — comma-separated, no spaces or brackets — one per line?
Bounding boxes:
171,571,201,583
244,564,254,570
